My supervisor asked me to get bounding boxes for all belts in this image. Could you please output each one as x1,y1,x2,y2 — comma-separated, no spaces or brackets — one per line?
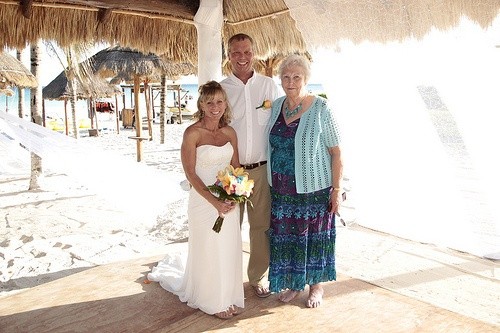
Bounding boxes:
240,161,267,170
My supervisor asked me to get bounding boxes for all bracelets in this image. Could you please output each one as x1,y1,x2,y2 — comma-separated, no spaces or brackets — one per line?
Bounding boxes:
331,187,342,191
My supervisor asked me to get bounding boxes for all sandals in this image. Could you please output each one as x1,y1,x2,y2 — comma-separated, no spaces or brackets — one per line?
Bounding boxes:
252,285,271,297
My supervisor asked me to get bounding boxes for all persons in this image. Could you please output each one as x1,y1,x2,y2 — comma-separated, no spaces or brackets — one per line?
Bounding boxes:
263,53,343,309
147,80,245,318
219,33,281,299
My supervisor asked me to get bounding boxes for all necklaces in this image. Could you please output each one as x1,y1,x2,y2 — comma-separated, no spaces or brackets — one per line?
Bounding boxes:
285,98,305,120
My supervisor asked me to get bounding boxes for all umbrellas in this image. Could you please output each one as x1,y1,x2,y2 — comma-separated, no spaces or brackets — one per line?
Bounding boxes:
43,40,198,163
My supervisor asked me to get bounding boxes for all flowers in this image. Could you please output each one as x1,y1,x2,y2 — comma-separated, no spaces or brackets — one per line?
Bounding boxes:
256,98,273,112
202,163,257,233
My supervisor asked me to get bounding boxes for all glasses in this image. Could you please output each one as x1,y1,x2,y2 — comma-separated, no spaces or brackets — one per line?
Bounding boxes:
336,205,358,226
